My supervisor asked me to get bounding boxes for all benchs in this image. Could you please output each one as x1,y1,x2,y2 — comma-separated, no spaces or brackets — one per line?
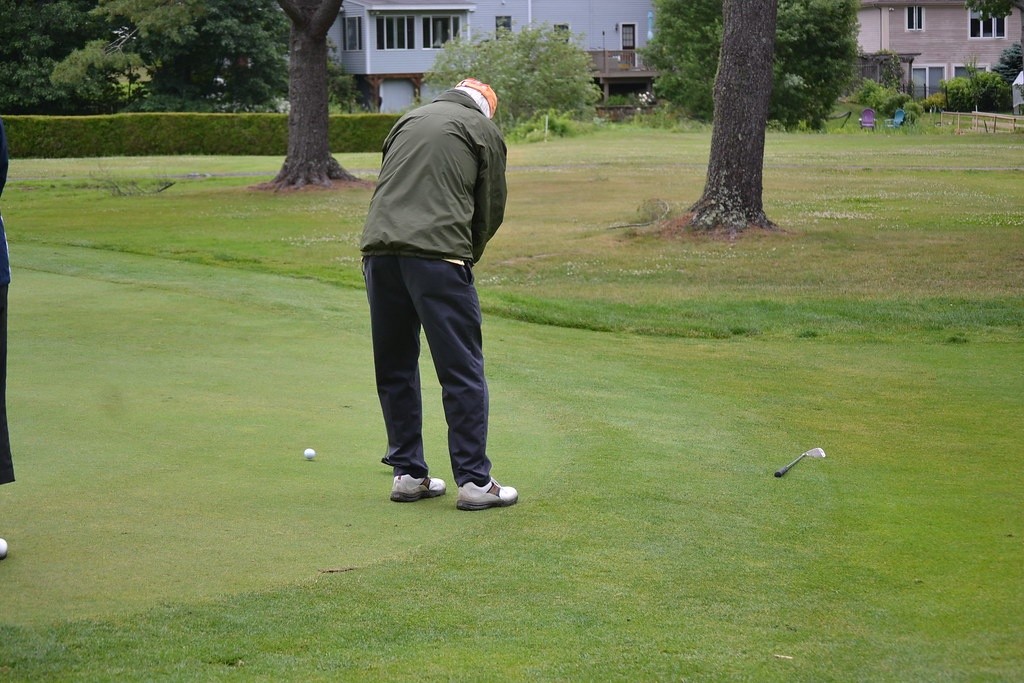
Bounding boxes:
971,110,1024,134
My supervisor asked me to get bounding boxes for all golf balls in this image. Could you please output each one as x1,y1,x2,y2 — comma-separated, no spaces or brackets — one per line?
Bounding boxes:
302,446,317,459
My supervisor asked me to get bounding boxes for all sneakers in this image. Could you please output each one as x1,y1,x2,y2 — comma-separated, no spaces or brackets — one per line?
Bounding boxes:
390,474,447,502
456,477,519,510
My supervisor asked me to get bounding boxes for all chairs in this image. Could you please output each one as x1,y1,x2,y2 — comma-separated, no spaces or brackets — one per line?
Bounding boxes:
883,108,905,129
858,108,876,132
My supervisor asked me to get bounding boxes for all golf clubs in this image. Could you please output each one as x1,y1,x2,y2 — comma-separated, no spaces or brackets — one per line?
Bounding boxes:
773,446,826,476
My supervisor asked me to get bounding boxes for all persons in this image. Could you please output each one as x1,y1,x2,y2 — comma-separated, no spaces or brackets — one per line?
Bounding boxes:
361,77,518,511
0,113,20,561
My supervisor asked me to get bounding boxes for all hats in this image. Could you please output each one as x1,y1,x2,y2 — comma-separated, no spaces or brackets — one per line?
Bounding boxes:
455,77,497,120
